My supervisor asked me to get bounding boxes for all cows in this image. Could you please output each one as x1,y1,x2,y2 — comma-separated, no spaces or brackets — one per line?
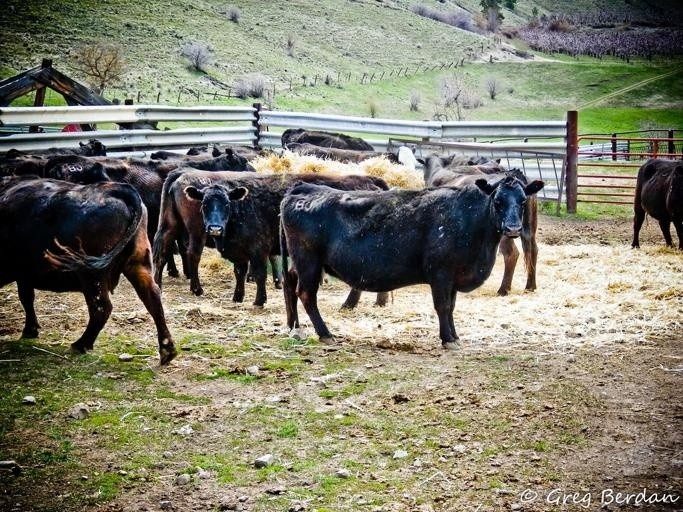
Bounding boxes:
630,158,683,251
0,128,546,365
